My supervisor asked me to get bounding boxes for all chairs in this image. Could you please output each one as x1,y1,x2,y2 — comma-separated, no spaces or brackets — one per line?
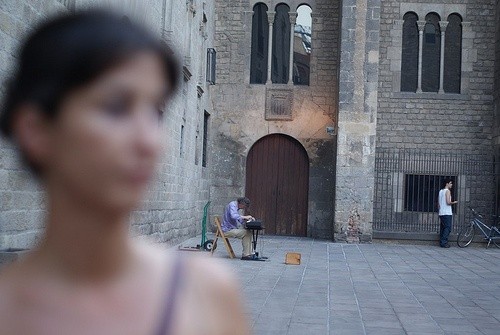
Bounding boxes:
210,215,236,258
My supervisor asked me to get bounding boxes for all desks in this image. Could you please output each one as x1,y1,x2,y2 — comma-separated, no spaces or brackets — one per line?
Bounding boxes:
245,228,266,261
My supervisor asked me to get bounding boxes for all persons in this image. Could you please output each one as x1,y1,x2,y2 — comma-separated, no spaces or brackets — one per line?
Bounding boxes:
0,9,251,335
221,197,260,260
438,179,458,248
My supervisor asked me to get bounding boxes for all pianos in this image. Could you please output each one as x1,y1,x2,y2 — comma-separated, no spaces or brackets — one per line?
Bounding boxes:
242,219,267,261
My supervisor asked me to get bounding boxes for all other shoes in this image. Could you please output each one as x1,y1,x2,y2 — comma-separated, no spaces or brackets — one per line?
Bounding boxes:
241,255,256,260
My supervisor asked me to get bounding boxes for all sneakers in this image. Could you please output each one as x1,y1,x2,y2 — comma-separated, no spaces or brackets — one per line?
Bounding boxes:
441,243,451,248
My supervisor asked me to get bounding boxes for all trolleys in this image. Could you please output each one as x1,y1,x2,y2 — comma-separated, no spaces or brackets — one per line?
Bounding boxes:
177,201,217,252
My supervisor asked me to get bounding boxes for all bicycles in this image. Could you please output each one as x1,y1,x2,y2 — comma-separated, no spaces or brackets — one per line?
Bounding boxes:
457,205,500,249
